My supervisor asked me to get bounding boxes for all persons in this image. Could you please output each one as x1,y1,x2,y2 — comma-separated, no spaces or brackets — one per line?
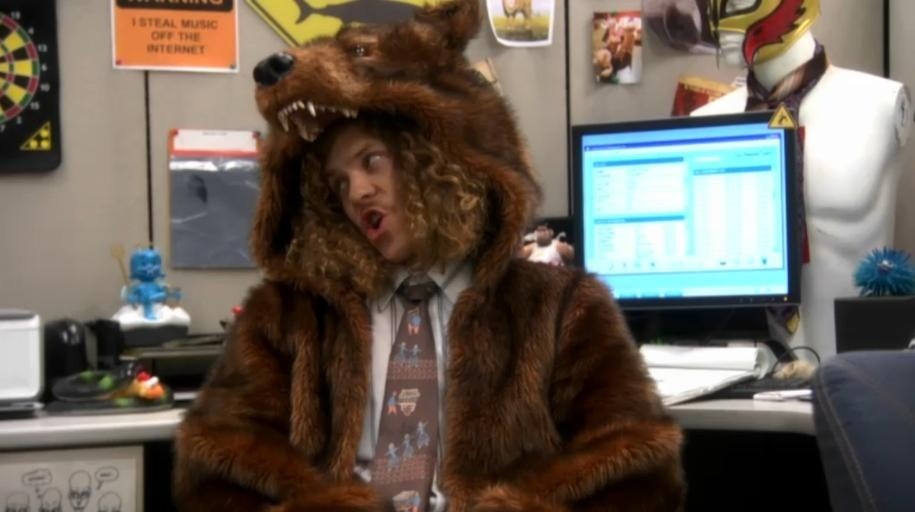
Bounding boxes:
678,0,913,364
171,1,689,512
521,217,575,266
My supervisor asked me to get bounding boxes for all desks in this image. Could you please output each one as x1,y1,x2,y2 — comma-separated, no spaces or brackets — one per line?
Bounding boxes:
1,347,817,512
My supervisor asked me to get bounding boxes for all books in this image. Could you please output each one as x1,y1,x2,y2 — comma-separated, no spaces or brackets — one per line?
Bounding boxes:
638,343,776,408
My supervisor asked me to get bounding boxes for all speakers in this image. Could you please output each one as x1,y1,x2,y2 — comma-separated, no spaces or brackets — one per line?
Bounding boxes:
834,295,915,353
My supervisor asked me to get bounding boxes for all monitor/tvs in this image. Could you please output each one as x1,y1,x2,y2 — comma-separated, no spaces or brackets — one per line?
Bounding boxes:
569,107,803,309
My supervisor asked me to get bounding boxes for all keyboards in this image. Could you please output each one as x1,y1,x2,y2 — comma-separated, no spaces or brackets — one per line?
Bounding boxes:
718,376,812,398
